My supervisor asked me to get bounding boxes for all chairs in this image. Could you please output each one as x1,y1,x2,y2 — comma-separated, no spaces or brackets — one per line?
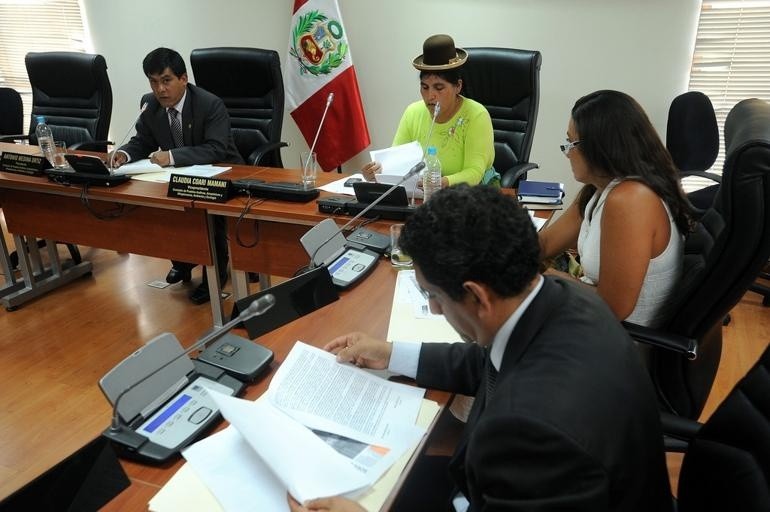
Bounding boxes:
456,47,543,189
666,93,769,326
189,47,288,283
621,95,770,447
0,51,115,279
453,337,769,510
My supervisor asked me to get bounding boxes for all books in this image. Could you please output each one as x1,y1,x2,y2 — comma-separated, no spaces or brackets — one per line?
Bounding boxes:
515,178,567,211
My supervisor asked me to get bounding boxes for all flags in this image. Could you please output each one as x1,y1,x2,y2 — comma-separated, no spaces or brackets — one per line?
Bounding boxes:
286,1,371,170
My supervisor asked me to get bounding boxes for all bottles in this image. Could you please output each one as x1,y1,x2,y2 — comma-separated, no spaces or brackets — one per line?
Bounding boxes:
36,116,58,167
421,147,443,203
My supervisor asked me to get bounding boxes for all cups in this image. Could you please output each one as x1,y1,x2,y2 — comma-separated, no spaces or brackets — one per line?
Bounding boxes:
48,141,69,169
297,150,318,185
388,222,415,272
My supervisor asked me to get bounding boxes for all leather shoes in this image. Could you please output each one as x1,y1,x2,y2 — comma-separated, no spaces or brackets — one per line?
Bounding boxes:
166,267,185,283
190,273,228,305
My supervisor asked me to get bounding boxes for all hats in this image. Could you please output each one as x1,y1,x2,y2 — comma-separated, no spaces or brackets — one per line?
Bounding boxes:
412,34,468,71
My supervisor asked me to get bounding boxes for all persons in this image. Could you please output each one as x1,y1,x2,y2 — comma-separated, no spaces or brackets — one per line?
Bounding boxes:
107,48,236,304
287,181,676,510
360,35,498,188
535,89,699,345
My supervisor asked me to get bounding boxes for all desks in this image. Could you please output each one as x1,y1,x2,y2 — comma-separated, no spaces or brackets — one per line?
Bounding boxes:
54,232,537,512
0,141,266,327
194,169,562,318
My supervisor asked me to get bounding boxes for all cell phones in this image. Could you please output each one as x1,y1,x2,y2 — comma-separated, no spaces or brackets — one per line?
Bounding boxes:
344,178,362,187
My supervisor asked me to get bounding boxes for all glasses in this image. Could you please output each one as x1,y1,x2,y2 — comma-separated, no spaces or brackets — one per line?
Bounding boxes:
560,140,586,157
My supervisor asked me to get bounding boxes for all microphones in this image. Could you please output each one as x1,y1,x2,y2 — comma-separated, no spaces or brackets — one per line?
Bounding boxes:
97,293,275,463
315,102,440,221
229,93,335,203
300,161,426,288
43,102,149,188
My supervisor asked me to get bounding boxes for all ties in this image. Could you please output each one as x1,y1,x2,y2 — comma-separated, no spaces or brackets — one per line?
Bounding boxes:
485,345,498,408
167,108,184,149
